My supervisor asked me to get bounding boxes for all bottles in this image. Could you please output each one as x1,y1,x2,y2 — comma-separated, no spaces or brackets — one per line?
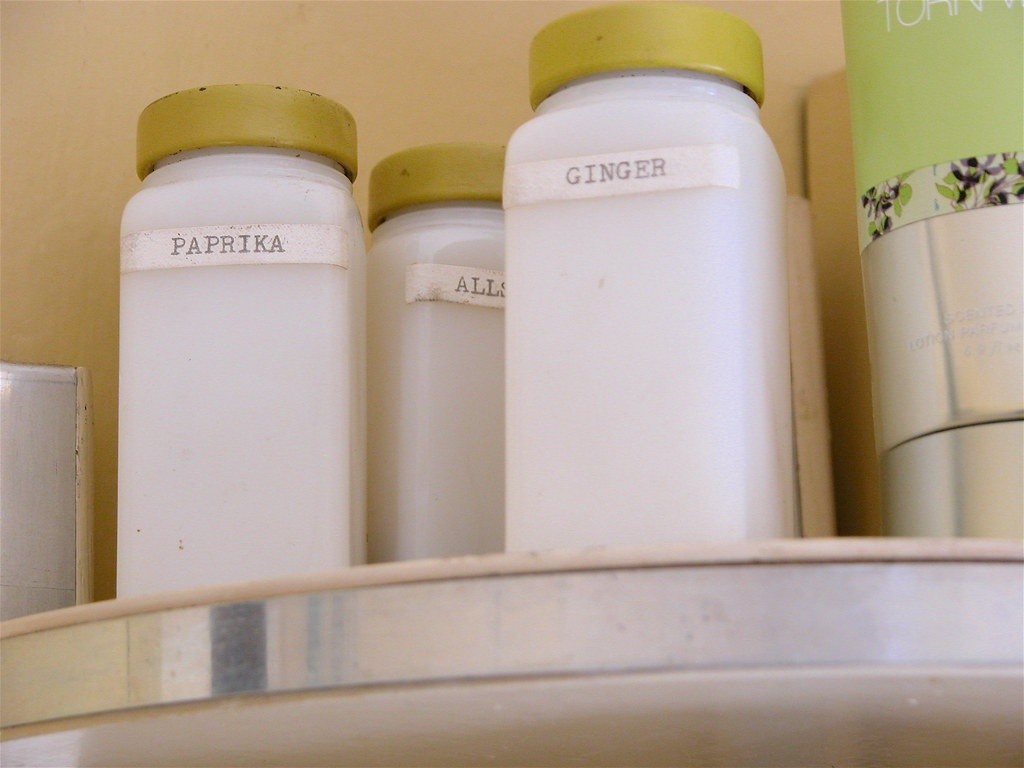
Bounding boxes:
114,84,366,597
498,0,787,553
369,141,503,559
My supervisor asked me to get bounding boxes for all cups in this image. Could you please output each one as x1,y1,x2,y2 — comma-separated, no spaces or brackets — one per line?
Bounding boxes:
843,0,1024,540
